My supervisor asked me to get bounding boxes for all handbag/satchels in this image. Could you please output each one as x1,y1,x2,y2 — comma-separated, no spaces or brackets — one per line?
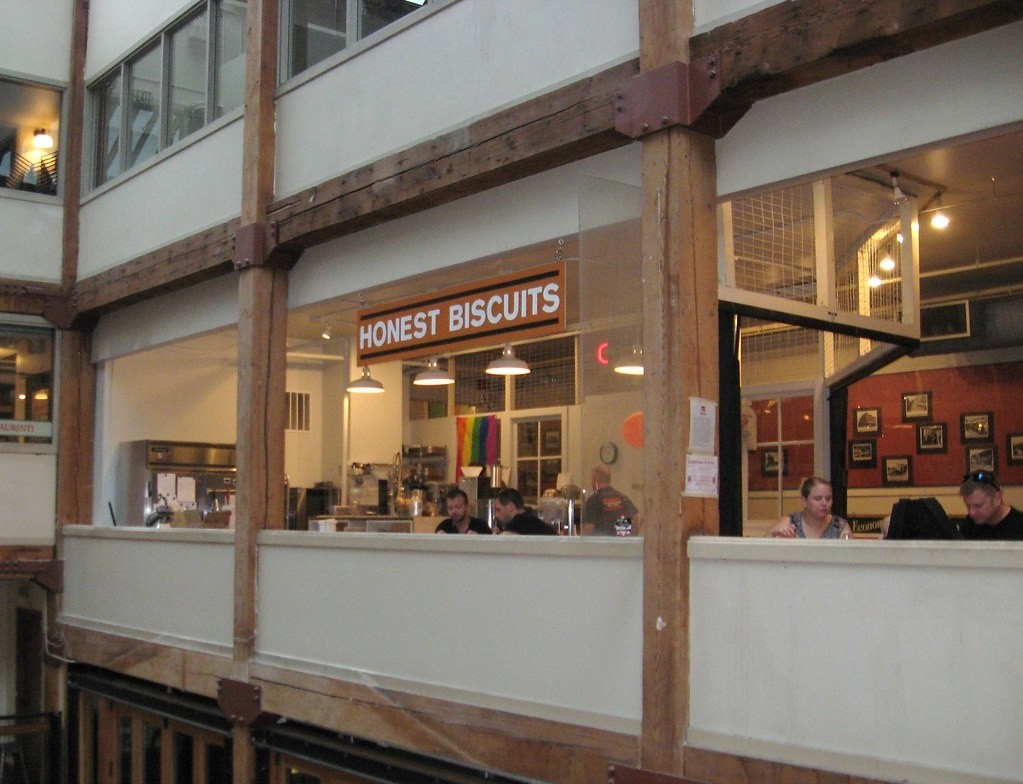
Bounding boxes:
886,498,962,540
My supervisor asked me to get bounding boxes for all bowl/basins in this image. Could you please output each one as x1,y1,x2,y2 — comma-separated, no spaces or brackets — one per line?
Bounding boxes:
461,467,483,477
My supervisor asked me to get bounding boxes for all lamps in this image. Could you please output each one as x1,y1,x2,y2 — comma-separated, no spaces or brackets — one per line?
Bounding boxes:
485,344,530,375
347,367,385,393
413,354,454,386
615,341,645,375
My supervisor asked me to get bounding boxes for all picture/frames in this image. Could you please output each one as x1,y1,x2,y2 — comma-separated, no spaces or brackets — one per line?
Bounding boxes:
546,429,561,452
848,439,877,469
917,423,947,453
763,448,788,477
901,391,932,422
854,406,882,437
1006,433,1023,465
960,410,992,443
881,453,912,485
967,445,999,475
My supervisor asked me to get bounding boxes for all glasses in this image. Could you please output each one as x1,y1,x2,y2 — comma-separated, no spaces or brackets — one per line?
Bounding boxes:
961,473,1000,491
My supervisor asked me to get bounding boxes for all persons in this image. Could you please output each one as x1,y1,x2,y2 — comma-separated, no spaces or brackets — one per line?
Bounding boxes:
959,468,1023,540
764,478,854,540
491,487,560,536
434,488,494,535
582,465,638,536
